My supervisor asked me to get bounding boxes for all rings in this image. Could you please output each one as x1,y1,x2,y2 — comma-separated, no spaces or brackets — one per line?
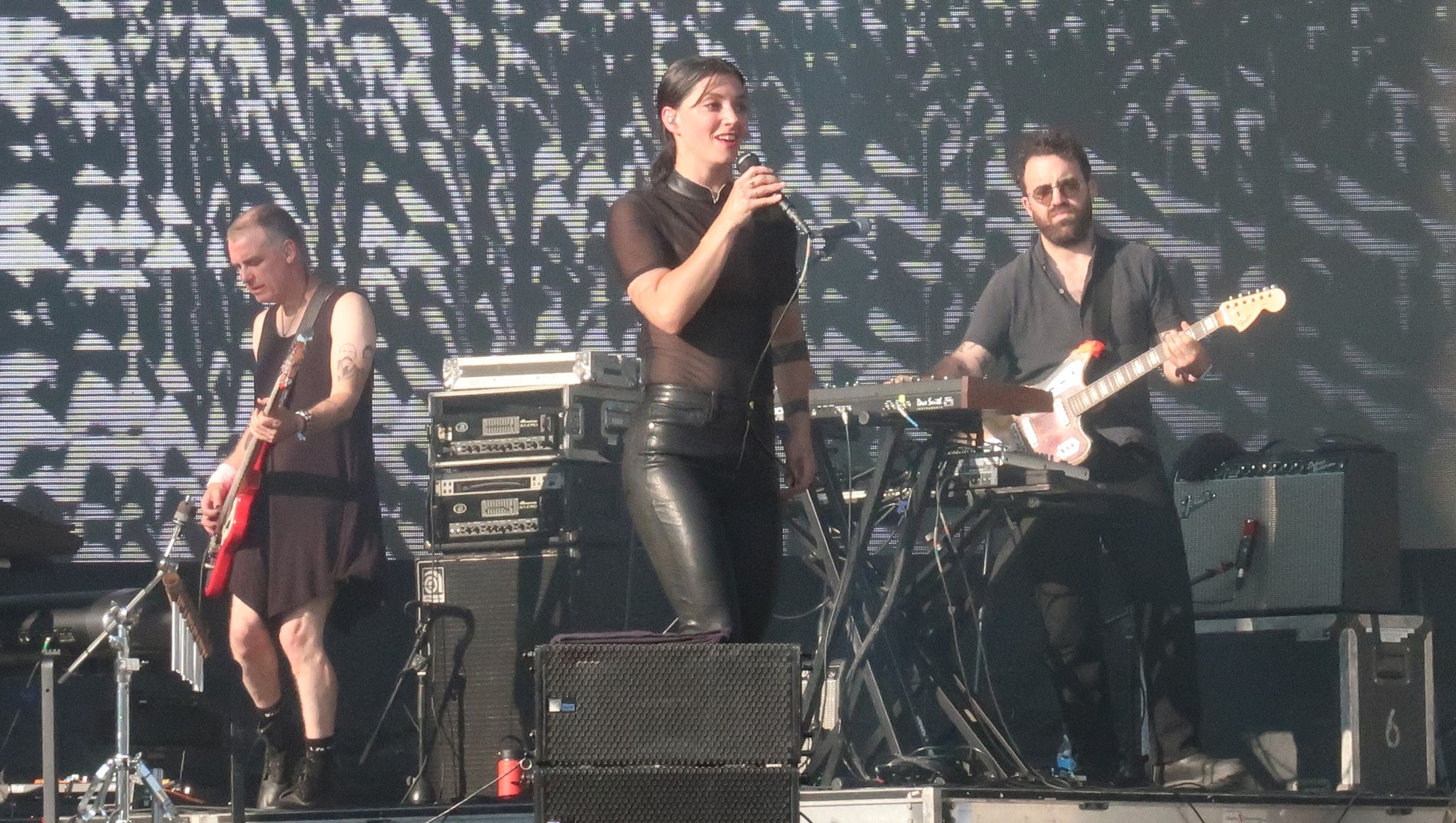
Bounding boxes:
749,178,756,188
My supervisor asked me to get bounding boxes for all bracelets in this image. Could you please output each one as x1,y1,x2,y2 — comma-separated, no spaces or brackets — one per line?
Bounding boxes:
1194,361,1213,382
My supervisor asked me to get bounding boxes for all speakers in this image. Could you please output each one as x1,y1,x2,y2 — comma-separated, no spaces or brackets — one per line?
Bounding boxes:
411,545,645,804
524,642,801,823
1173,449,1405,613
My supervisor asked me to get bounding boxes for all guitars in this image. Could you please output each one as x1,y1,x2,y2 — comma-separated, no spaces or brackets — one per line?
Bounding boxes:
202,333,308,599
957,284,1286,467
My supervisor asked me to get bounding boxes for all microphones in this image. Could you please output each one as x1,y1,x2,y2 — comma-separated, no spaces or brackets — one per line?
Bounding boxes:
172,497,194,527
1237,518,1256,585
404,601,470,618
736,152,809,235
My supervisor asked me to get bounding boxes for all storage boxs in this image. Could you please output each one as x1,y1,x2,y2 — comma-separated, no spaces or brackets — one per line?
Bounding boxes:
1184,612,1442,792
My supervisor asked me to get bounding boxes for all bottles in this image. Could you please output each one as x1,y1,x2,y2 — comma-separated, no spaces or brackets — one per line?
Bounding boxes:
1055,734,1077,774
497,748,522,799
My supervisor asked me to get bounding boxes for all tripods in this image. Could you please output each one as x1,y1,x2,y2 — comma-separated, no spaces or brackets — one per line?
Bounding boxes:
54,522,184,823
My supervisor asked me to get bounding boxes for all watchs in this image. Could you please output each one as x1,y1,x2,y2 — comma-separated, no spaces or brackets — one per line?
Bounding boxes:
781,399,809,417
296,411,314,443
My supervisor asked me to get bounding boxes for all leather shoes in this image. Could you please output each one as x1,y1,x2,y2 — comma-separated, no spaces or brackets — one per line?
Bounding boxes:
1162,753,1247,793
1072,764,1110,789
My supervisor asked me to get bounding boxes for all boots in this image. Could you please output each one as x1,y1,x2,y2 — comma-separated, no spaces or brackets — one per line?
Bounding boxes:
276,753,353,811
256,695,302,810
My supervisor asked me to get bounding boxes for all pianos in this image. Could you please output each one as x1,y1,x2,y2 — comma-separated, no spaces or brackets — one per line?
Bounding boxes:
765,372,1054,790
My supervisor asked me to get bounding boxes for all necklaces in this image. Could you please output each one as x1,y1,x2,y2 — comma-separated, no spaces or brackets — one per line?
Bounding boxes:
281,263,308,338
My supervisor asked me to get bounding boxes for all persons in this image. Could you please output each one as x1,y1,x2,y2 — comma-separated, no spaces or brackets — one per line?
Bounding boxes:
201,203,384,810
606,56,818,639
883,129,1248,794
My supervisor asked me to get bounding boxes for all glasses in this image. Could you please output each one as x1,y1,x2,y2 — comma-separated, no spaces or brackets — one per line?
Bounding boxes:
1026,176,1086,205
